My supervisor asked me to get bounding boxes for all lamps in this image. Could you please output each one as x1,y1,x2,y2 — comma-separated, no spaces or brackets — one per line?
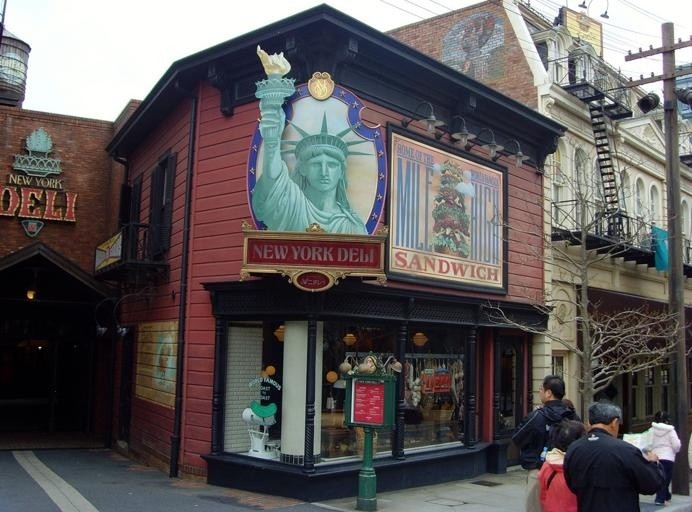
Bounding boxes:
636,87,692,114
402,99,529,168
564,0,610,21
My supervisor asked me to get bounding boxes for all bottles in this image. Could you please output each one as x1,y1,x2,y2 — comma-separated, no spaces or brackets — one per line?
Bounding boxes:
540,446,548,462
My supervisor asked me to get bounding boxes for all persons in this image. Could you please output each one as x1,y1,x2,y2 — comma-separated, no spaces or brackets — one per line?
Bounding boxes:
646,409,683,505
511,375,582,511
537,418,588,512
250,43,372,236
562,398,666,511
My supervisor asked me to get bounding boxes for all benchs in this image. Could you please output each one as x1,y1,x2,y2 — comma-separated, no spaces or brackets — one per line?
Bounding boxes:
319,423,435,458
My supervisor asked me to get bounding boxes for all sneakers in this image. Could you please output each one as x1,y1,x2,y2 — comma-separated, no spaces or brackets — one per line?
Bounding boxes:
654,499,671,506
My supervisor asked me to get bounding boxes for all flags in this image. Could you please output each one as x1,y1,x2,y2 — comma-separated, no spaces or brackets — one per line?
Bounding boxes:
651,227,671,274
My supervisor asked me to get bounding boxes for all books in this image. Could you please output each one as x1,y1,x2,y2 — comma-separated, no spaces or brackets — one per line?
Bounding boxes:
619,426,655,462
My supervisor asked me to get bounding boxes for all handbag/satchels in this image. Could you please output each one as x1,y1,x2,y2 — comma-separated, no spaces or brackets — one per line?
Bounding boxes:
518,408,553,471
